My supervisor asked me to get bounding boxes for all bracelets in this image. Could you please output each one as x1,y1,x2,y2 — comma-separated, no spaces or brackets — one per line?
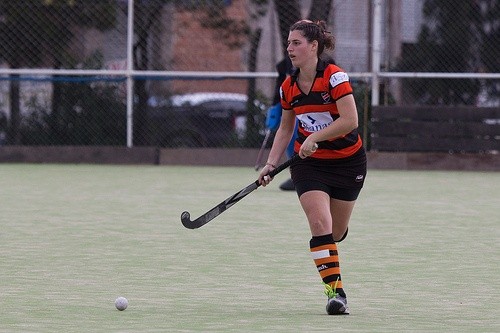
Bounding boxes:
266,163,276,169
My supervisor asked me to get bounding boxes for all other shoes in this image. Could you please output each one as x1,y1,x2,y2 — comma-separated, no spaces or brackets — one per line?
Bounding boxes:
279,178,294,189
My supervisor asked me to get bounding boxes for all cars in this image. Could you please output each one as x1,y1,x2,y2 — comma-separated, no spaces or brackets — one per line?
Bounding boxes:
152,91,270,140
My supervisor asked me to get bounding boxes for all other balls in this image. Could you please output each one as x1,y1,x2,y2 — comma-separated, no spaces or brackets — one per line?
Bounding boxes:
115,297,129,311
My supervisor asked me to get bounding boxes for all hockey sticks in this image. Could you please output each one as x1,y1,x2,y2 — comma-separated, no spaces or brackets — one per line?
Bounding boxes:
256,128,271,170
180,143,318,228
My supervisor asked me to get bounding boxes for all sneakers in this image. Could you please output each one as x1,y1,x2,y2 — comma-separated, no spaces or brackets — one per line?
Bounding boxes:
320,277,349,314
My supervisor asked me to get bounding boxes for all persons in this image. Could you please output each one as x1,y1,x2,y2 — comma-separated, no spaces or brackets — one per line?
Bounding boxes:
258,21,367,314
273,20,336,191
